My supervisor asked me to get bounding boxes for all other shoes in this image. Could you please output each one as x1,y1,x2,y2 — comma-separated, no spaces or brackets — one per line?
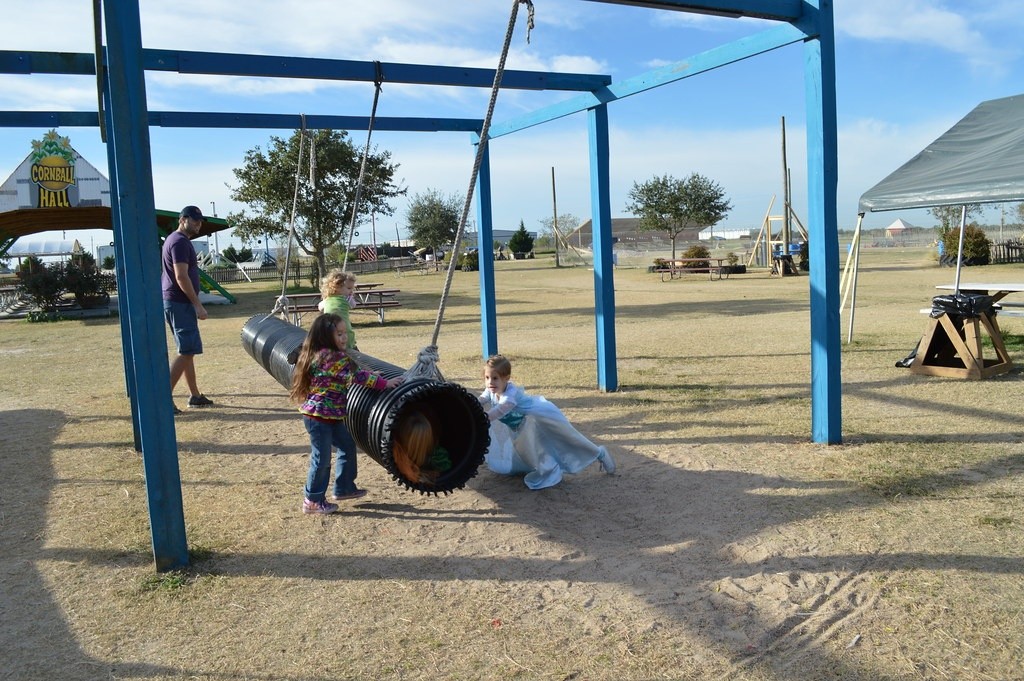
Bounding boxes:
288,342,304,365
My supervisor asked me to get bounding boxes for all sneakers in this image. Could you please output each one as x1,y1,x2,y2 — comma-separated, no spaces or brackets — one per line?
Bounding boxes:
333,486,367,500
597,445,616,474
302,496,339,513
189,394,213,406
173,400,181,414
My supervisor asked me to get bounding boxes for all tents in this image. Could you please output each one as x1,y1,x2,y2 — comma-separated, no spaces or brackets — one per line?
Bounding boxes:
847,94,1024,345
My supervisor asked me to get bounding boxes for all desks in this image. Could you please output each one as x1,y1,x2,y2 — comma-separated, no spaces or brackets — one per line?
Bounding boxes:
353,283,383,302
275,289,400,320
936,283,1024,305
393,264,423,268
661,258,728,278
433,261,450,263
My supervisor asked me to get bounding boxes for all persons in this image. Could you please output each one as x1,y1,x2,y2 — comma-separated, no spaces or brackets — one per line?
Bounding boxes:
476,354,615,489
316,269,360,351
390,408,451,479
162,206,214,415
289,314,405,513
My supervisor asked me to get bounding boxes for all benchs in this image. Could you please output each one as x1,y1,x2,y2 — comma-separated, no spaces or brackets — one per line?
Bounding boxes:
656,268,729,283
271,301,402,326
366,292,396,301
393,260,450,277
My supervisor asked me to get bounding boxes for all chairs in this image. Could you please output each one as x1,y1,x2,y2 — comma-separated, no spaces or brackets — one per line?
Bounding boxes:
919,300,1024,318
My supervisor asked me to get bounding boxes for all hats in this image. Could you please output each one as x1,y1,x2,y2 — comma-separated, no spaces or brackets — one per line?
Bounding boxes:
179,206,208,221
